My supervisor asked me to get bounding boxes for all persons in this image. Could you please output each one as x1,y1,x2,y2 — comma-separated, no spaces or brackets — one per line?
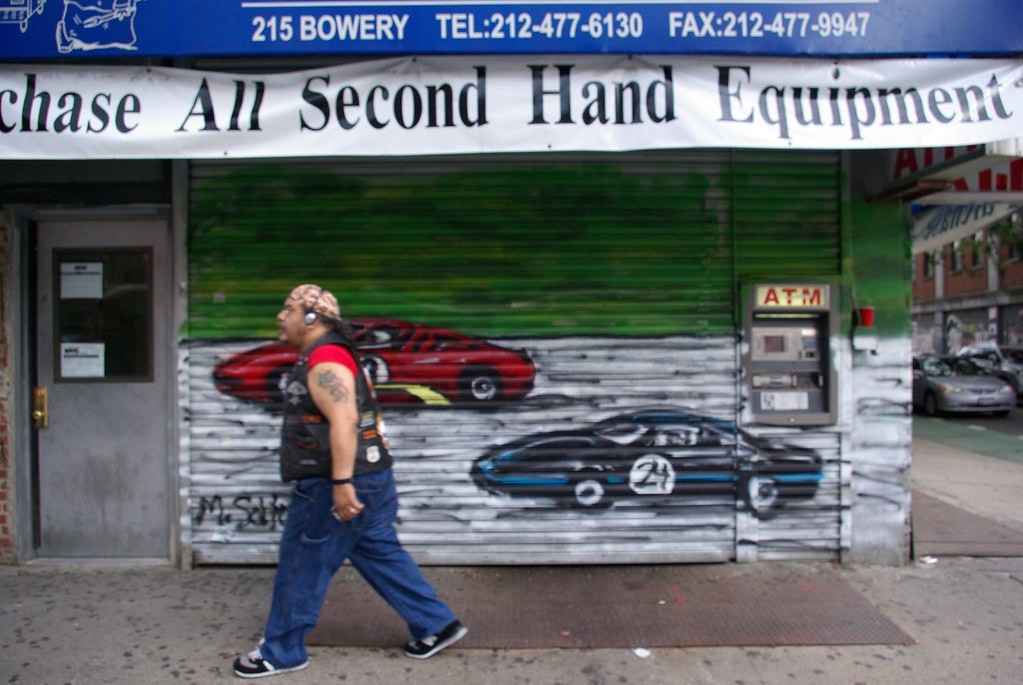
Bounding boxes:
232,283,469,679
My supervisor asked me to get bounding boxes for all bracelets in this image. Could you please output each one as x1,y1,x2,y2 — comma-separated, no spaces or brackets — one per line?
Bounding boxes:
330,479,352,485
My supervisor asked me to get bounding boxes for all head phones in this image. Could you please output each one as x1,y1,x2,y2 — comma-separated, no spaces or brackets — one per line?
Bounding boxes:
304,288,325,325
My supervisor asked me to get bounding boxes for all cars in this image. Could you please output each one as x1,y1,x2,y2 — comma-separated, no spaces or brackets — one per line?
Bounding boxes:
913,356,1017,417
956,341,1023,392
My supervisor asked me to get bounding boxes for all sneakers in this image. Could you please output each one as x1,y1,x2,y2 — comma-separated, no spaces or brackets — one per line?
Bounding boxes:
405,619,467,659
232,640,311,677
